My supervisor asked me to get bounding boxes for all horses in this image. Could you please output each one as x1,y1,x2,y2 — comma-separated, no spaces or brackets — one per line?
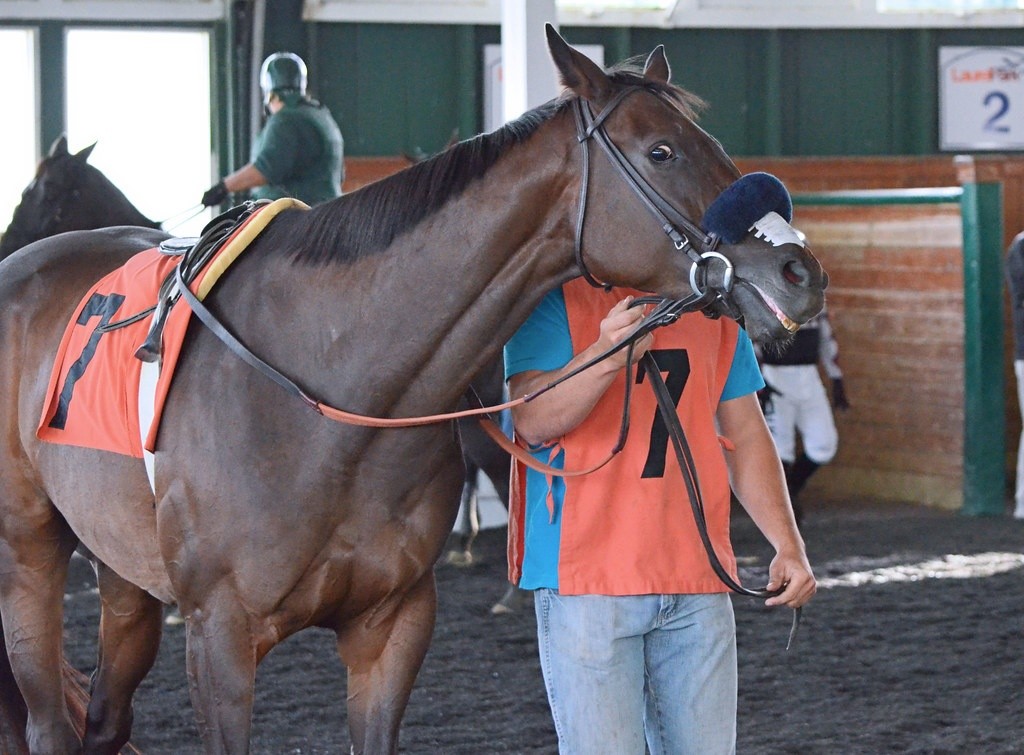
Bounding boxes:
0,23,831,754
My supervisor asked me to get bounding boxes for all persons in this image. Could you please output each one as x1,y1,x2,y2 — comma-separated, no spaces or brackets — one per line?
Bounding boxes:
199,49,345,214
748,308,853,526
1000,230,1024,522
505,272,819,755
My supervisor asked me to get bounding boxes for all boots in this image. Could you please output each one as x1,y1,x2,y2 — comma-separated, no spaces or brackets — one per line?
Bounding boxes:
780,453,821,529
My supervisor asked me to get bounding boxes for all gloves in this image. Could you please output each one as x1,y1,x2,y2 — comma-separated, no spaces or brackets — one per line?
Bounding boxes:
757,379,784,416
201,181,227,208
831,379,847,410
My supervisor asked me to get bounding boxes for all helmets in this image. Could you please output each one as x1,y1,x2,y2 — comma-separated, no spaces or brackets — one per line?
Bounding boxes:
260,52,308,89
